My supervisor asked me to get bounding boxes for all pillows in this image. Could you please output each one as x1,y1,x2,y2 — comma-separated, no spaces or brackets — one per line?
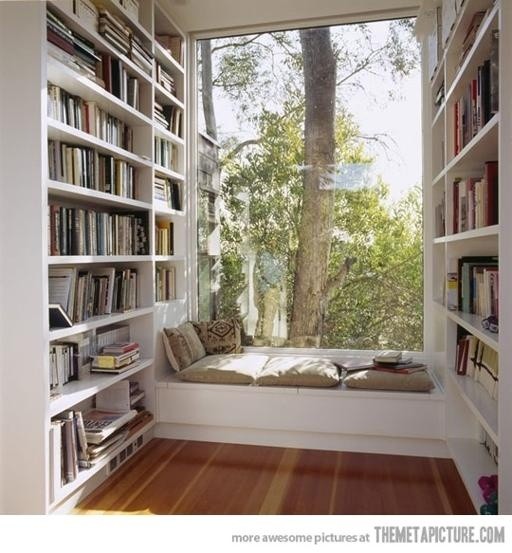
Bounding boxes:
190,316,243,355
342,367,436,391
162,322,206,371
256,356,339,387
175,352,269,384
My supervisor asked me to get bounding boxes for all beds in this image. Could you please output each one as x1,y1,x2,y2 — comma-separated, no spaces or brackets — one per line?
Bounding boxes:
156,347,447,457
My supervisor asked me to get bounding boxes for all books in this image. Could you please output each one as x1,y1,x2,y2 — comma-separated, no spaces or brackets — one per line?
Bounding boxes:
46,0,184,503
434,0,499,470
334,351,427,373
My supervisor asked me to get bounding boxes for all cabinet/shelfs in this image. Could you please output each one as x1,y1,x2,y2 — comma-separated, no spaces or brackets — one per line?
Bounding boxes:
427,0,502,517
153,0,188,306
45,0,154,509
